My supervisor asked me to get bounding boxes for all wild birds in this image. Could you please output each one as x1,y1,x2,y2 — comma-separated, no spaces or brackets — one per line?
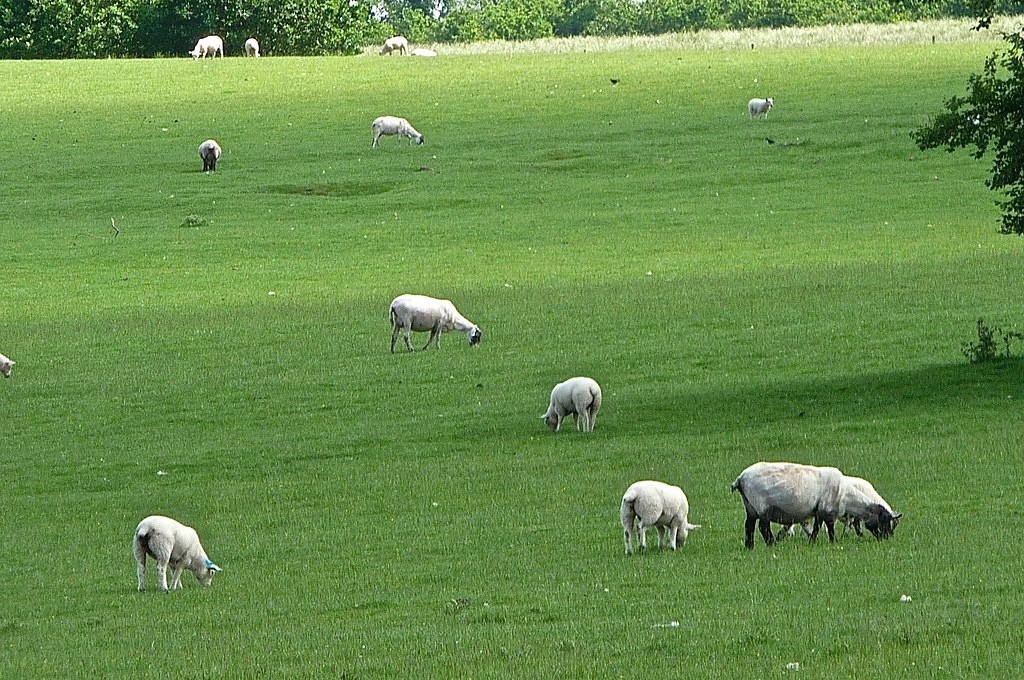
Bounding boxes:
765,137,775,145
610,78,620,85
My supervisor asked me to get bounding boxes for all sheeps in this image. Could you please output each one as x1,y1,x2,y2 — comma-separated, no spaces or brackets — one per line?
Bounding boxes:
0,353,16,378
371,116,424,148
188,35,224,61
378,36,408,57
245,38,260,58
387,294,483,354
411,48,437,56
133,515,223,593
620,480,702,556
748,97,774,121
198,139,222,175
540,376,603,433
730,461,903,550
